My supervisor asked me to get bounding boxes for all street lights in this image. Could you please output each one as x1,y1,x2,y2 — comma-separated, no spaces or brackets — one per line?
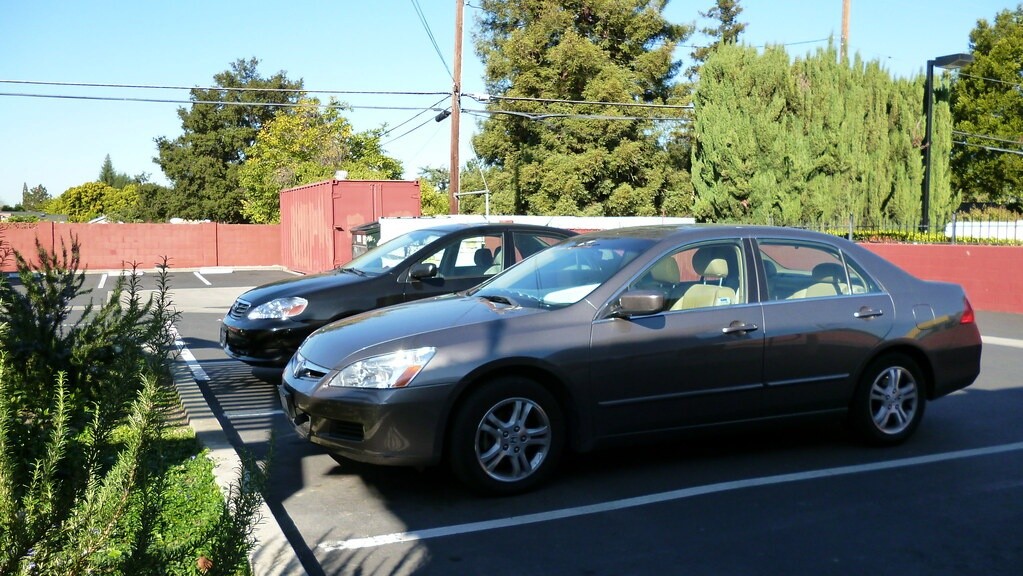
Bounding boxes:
919,54,975,233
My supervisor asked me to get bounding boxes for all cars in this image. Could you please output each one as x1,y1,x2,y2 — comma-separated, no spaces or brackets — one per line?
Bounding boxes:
219,221,653,371
277,223,984,500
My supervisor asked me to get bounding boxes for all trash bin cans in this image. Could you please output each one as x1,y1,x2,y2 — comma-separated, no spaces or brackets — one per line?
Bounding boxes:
350,221,383,268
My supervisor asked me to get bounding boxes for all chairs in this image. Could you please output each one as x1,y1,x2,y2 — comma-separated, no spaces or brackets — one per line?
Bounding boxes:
640,254,682,313
461,246,503,275
669,247,737,311
761,260,863,299
